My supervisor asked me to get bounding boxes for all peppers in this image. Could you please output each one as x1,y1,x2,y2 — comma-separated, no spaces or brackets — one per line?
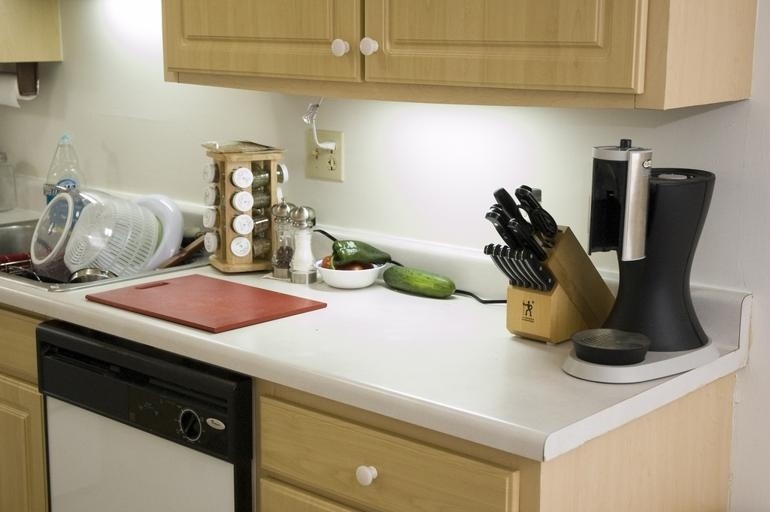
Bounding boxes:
332,240,391,267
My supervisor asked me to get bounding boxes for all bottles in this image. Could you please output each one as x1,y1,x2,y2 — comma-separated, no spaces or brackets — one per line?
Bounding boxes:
272,196,299,281
199,154,291,263
0,150,17,213
42,133,87,226
288,203,321,283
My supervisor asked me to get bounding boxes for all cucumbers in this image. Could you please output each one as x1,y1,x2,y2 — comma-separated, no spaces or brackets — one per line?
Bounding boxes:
383,265,456,298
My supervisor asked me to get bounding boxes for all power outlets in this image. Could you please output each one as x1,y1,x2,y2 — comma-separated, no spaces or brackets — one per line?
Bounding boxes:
303,129,346,182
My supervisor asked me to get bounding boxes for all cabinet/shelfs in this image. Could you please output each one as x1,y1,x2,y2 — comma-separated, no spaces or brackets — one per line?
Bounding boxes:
250,374,735,512
161,0,759,112
0,303,58,512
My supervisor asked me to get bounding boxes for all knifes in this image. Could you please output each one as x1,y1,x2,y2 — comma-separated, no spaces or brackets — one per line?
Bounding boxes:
482,183,561,292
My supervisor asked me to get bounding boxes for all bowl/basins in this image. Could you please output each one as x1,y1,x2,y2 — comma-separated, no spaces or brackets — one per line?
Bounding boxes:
29,185,186,288
313,257,388,290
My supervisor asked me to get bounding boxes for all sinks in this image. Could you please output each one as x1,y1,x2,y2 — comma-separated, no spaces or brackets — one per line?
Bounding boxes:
0,219,211,292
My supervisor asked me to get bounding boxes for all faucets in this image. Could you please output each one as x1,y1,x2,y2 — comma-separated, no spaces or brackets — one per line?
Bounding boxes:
43,183,98,212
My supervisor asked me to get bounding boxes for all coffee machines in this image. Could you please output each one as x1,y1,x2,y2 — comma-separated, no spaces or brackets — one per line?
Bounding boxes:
561,139,721,385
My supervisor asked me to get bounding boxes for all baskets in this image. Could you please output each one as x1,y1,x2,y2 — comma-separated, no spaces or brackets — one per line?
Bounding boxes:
62,199,159,279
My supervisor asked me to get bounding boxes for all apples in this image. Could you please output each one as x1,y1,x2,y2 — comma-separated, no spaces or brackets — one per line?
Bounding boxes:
347,263,368,270
322,256,331,269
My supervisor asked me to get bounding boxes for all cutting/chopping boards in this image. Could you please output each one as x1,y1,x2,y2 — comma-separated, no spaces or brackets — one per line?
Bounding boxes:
84,272,328,334
